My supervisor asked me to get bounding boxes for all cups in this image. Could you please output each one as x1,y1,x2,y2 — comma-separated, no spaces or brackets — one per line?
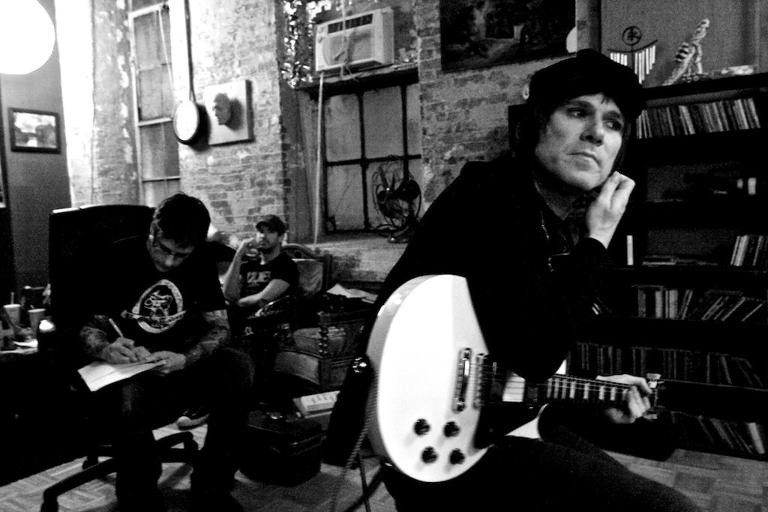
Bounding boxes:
27,308,46,339
3,304,22,335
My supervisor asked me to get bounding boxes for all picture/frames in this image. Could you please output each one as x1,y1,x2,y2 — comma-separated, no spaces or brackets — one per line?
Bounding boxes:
7,107,61,154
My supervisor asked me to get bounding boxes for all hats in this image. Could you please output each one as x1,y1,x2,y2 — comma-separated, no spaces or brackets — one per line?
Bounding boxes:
529,56,645,118
255,214,286,234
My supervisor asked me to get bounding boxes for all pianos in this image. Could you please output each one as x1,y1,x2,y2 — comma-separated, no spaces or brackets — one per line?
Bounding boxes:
293,391,339,420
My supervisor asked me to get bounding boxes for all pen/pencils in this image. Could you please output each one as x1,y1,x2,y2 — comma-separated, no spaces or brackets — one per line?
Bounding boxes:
109,317,141,362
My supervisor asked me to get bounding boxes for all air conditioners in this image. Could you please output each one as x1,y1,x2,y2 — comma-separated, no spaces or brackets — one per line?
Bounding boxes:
314,6,395,75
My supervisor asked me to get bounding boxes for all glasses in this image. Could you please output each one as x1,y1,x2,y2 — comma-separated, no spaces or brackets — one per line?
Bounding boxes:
158,241,188,258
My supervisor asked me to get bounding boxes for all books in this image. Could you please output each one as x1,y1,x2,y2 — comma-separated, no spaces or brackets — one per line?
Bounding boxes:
630,285,765,322
730,234,765,267
636,99,760,139
578,342,763,388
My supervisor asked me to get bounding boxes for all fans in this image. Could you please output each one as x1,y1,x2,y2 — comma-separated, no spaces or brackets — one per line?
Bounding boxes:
372,155,422,244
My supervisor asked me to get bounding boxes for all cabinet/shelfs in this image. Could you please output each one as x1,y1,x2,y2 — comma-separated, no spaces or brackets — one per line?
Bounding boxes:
509,71,768,464
0,348,55,487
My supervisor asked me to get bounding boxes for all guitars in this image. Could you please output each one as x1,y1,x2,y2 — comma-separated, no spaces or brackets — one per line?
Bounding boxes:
362,274,666,483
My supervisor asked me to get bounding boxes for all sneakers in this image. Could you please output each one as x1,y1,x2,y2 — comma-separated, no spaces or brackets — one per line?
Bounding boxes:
176,406,210,429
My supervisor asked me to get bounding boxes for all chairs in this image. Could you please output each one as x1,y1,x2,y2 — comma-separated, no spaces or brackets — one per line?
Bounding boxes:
255,279,384,392
38,203,202,511
241,244,333,352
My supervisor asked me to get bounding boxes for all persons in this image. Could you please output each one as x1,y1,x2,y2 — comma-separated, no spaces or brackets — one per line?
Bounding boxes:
348,50,703,510
172,213,307,429
53,191,257,512
